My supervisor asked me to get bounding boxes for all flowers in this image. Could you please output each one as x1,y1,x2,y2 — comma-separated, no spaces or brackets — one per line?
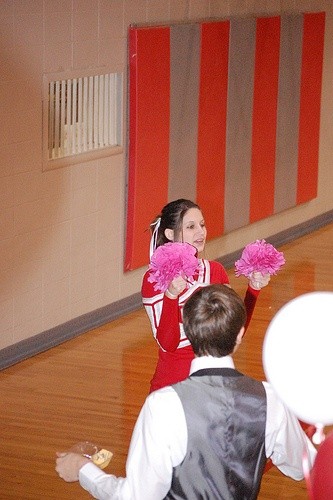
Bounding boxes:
147,241,203,294
234,239,287,282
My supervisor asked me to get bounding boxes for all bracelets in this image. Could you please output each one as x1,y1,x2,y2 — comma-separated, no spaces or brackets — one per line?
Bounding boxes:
166,289,179,298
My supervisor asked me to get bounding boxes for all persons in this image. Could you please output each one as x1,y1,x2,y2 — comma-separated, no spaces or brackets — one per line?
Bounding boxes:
140,198,272,395
55,283,318,500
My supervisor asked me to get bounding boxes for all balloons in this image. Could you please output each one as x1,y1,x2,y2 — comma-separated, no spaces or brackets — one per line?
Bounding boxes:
262,291,333,444
